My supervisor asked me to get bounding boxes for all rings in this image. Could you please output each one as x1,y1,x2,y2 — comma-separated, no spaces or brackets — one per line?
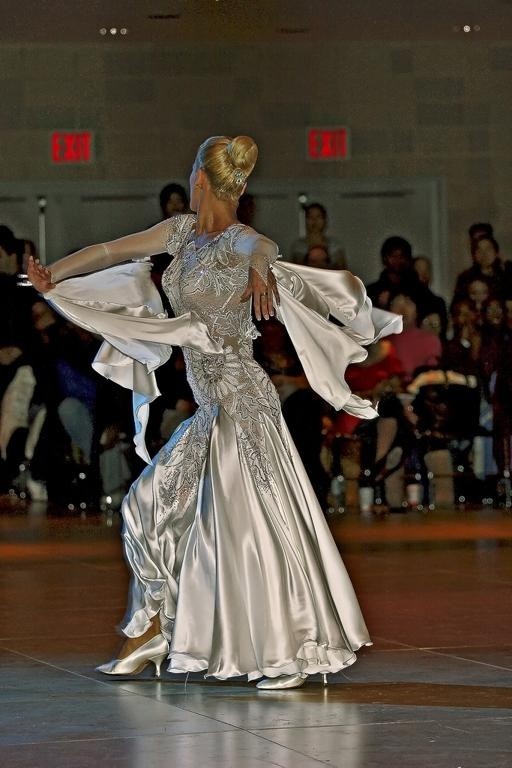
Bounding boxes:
260,291,267,296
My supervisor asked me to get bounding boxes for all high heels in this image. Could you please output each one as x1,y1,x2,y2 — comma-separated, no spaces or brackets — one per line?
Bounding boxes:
256,672,329,690
94,633,170,676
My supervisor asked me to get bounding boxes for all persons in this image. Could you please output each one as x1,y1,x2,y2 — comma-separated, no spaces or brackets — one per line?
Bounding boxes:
237,194,510,516
1,183,196,514
28,134,407,694
